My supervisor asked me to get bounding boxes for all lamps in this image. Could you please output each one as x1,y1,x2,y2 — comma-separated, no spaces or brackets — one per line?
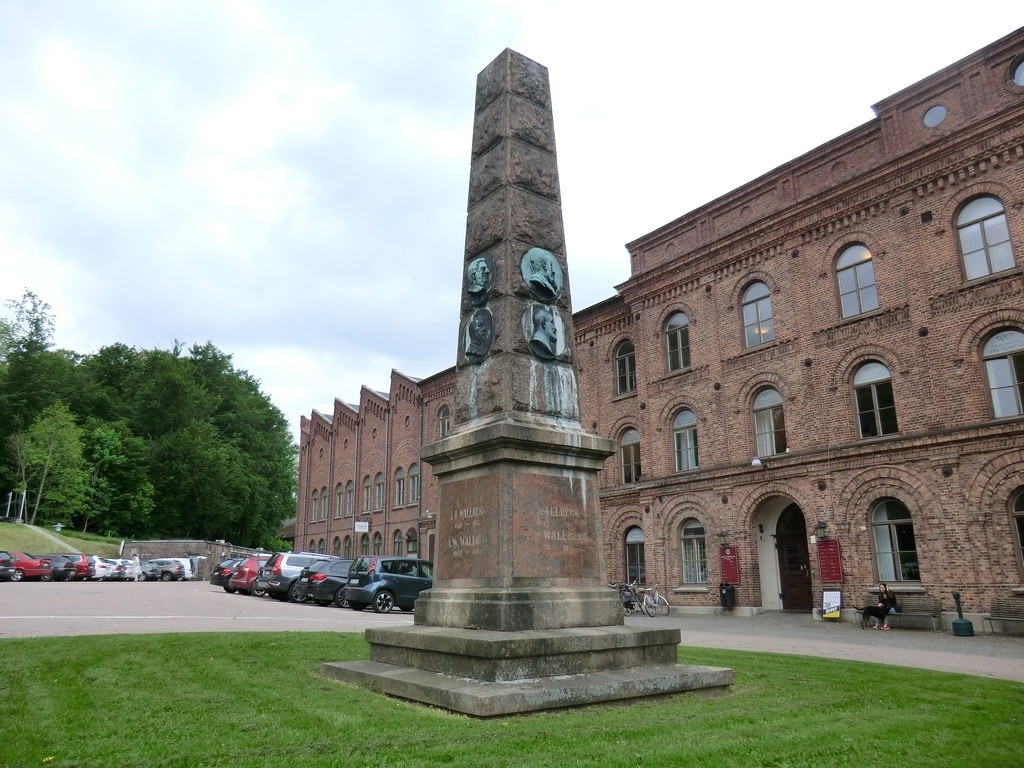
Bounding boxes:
750,456,767,471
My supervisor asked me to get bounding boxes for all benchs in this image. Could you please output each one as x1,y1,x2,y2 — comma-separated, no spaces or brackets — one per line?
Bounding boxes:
881,595,942,632
981,599,1024,636
620,589,645,611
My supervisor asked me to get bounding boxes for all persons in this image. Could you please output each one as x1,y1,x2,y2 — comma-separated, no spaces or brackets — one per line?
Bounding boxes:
465,315,487,357
467,259,491,294
529,251,561,298
854,583,896,631
529,308,559,357
131,554,140,582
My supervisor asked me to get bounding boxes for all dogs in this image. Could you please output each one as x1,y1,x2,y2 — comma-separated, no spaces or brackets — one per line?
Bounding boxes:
853,603,892,630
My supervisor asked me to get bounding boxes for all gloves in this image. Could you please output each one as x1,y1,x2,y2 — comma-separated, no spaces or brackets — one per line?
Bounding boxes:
878,603,884,607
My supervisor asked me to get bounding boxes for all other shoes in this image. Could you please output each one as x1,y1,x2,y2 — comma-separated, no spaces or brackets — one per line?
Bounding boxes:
881,624,890,630
873,624,880,629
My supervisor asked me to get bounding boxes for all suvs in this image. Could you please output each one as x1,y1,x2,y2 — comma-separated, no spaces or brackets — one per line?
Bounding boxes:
0,549,95,581
209,551,327,604
298,557,435,613
94,557,198,582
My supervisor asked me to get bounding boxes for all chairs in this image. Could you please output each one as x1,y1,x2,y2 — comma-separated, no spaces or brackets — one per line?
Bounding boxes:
400,564,414,576
383,564,389,572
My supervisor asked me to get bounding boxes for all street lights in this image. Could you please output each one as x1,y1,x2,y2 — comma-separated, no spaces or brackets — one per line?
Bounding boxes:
52,522,66,553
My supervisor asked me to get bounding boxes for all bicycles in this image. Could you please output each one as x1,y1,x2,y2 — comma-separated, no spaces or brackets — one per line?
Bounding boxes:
607,578,671,618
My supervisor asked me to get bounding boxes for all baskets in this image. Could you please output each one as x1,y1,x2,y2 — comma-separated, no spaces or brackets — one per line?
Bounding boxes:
628,585,640,595
